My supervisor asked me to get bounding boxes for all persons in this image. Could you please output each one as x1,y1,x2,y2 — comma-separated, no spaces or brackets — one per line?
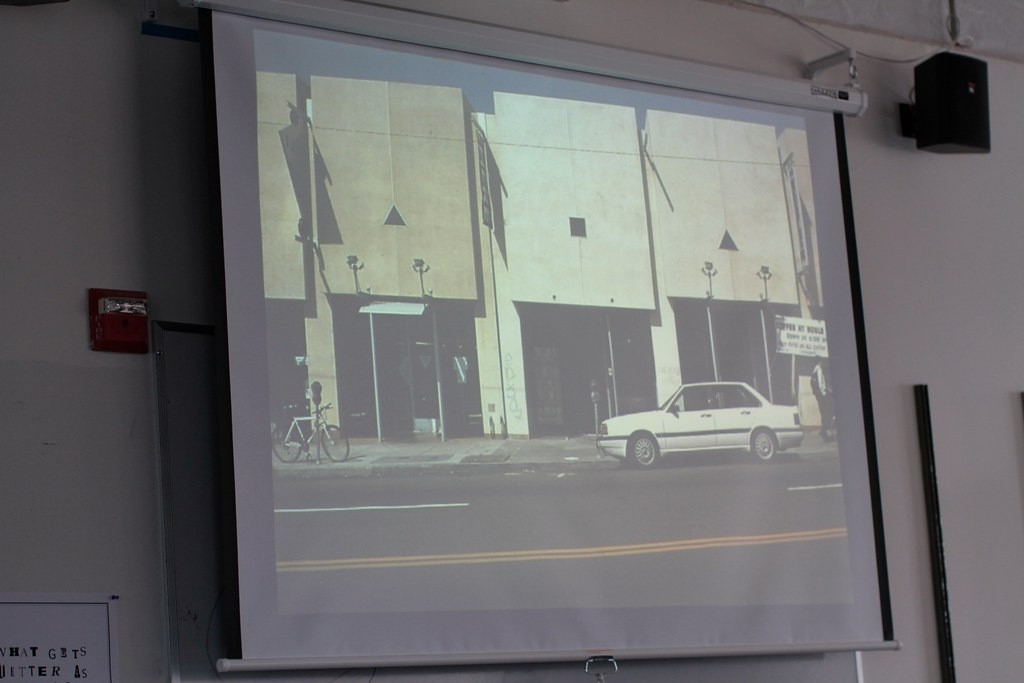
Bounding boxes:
810,355,832,442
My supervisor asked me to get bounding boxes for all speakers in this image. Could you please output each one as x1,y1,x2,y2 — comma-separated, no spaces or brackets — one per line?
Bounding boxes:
914,52,994,155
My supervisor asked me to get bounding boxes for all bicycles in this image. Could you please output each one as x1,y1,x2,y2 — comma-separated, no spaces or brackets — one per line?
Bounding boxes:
272,402,350,463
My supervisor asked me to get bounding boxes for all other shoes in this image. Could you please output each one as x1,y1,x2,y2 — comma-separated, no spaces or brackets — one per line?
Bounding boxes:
819,430,829,440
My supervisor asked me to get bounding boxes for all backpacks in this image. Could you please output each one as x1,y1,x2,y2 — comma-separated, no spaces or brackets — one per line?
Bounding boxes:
810,372,821,399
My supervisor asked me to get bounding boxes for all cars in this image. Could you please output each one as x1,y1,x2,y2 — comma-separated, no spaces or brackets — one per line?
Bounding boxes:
597,380,804,470
